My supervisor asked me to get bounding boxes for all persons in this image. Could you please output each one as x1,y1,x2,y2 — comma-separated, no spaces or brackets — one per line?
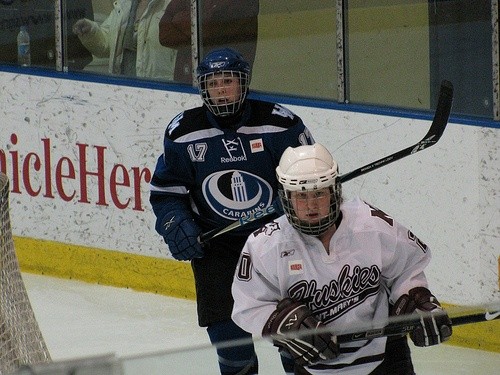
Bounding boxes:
72,0,178,83
231,144,453,375
0,0,95,71
149,45,316,375
159,0,260,88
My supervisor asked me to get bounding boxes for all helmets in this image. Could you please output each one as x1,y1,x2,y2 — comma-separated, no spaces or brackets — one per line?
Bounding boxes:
276,143,342,236
198,49,250,115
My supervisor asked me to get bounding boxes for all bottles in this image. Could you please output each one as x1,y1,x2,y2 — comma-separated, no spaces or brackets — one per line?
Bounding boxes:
17,26,32,67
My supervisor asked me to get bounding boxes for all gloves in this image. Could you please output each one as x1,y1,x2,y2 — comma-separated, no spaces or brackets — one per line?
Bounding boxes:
391,287,452,347
152,197,208,260
271,302,341,366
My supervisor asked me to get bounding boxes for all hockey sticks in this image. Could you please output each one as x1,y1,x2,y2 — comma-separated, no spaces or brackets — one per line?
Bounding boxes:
331,310,500,344
197,79,455,242
